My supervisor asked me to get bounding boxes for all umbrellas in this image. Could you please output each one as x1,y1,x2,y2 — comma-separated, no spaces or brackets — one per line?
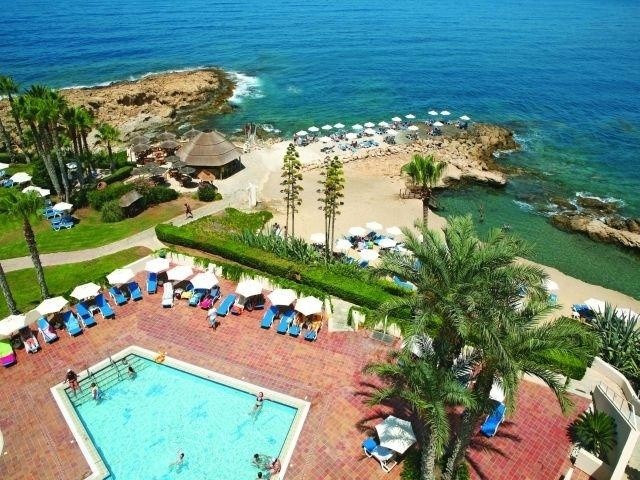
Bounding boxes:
402,335,435,360
291,104,474,147
0,162,10,169
66,162,77,170
449,351,471,380
10,171,51,200
488,374,509,402
131,128,203,176
52,202,74,211
376,414,416,454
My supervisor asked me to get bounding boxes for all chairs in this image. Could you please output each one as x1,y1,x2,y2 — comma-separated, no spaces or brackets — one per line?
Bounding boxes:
148,259,325,342
0,170,12,188
362,437,398,473
0,265,143,368
43,204,75,231
320,230,420,292
480,401,508,438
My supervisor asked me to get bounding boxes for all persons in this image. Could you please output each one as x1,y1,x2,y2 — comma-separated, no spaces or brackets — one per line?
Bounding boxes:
90,383,101,400
184,203,193,219
127,366,137,377
206,304,217,332
273,222,279,235
283,226,288,241
22,331,37,349
166,453,186,474
249,450,281,480
245,391,271,420
297,116,468,151
63,368,83,397
